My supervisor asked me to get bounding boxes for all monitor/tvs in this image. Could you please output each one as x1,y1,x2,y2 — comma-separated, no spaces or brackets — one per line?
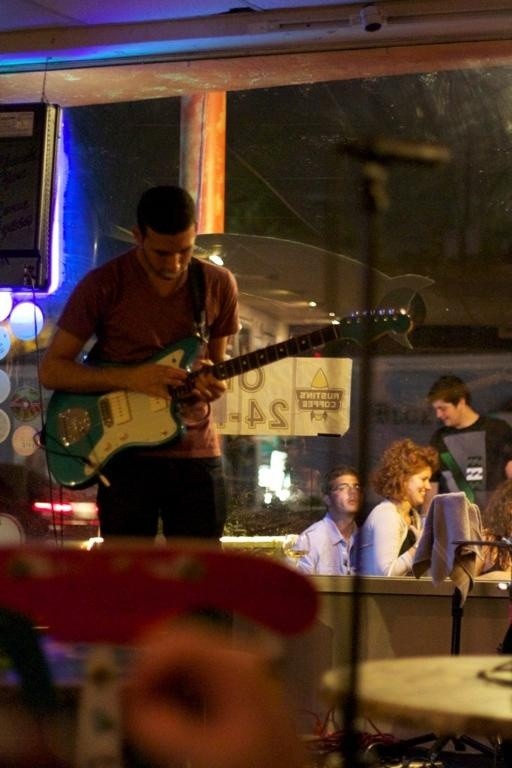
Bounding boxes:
0,102,65,296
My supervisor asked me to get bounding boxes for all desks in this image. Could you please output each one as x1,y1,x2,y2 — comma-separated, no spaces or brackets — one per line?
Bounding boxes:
324,653,510,768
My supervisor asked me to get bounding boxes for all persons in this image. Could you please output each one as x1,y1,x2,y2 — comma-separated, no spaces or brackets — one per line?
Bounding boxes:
481,478,512,572
0,464,85,716
426,373,512,515
291,465,363,575
37,183,243,652
360,434,442,579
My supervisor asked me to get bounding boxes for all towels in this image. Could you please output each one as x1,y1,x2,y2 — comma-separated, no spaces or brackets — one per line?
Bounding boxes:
410,490,485,608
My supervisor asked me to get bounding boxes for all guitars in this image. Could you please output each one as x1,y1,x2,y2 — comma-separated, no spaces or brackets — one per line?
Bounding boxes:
40,306,414,491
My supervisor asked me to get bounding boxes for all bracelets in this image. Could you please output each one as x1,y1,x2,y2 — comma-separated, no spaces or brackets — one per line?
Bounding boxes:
411,542,422,551
